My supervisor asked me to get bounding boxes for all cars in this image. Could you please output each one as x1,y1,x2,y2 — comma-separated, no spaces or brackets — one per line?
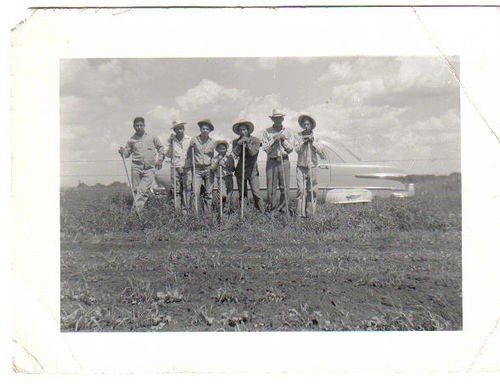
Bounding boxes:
201,135,416,203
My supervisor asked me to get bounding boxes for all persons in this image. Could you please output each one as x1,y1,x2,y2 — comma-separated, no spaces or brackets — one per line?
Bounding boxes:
261,107,294,221
117,116,168,218
163,120,192,210
181,118,216,216
293,112,327,220
209,137,237,215
230,118,267,218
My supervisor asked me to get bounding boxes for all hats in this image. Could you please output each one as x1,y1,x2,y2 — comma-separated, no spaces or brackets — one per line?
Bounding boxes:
232,121,255,135
171,121,187,129
299,115,316,129
269,109,285,117
214,140,229,150
197,120,214,131
